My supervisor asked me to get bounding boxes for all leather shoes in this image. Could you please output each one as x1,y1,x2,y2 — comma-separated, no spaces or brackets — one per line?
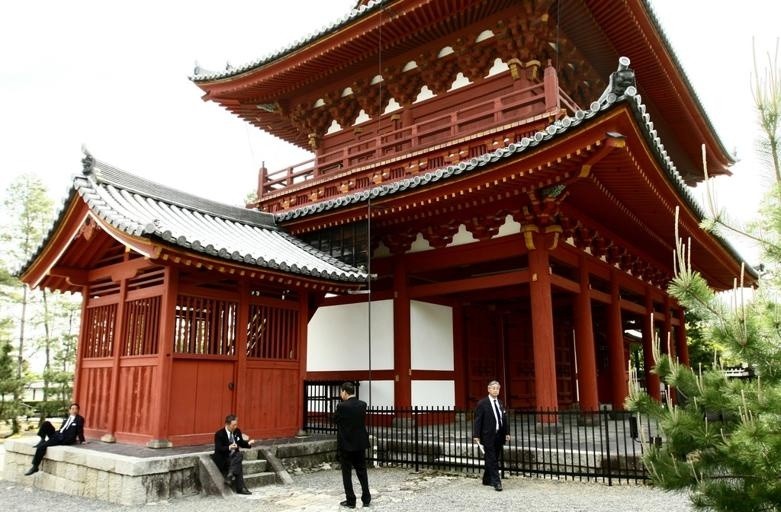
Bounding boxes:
490,483,502,491
33,440,45,448
341,501,355,508
363,504,369,507
225,476,232,485
237,487,252,495
24,467,39,476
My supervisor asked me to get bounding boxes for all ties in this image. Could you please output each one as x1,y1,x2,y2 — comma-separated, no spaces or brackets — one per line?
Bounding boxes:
62,416,71,433
494,400,502,430
229,432,233,443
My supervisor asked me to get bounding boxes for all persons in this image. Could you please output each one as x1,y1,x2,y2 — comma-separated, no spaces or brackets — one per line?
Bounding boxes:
24,402,86,476
214,412,257,494
473,379,514,492
329,383,374,508
